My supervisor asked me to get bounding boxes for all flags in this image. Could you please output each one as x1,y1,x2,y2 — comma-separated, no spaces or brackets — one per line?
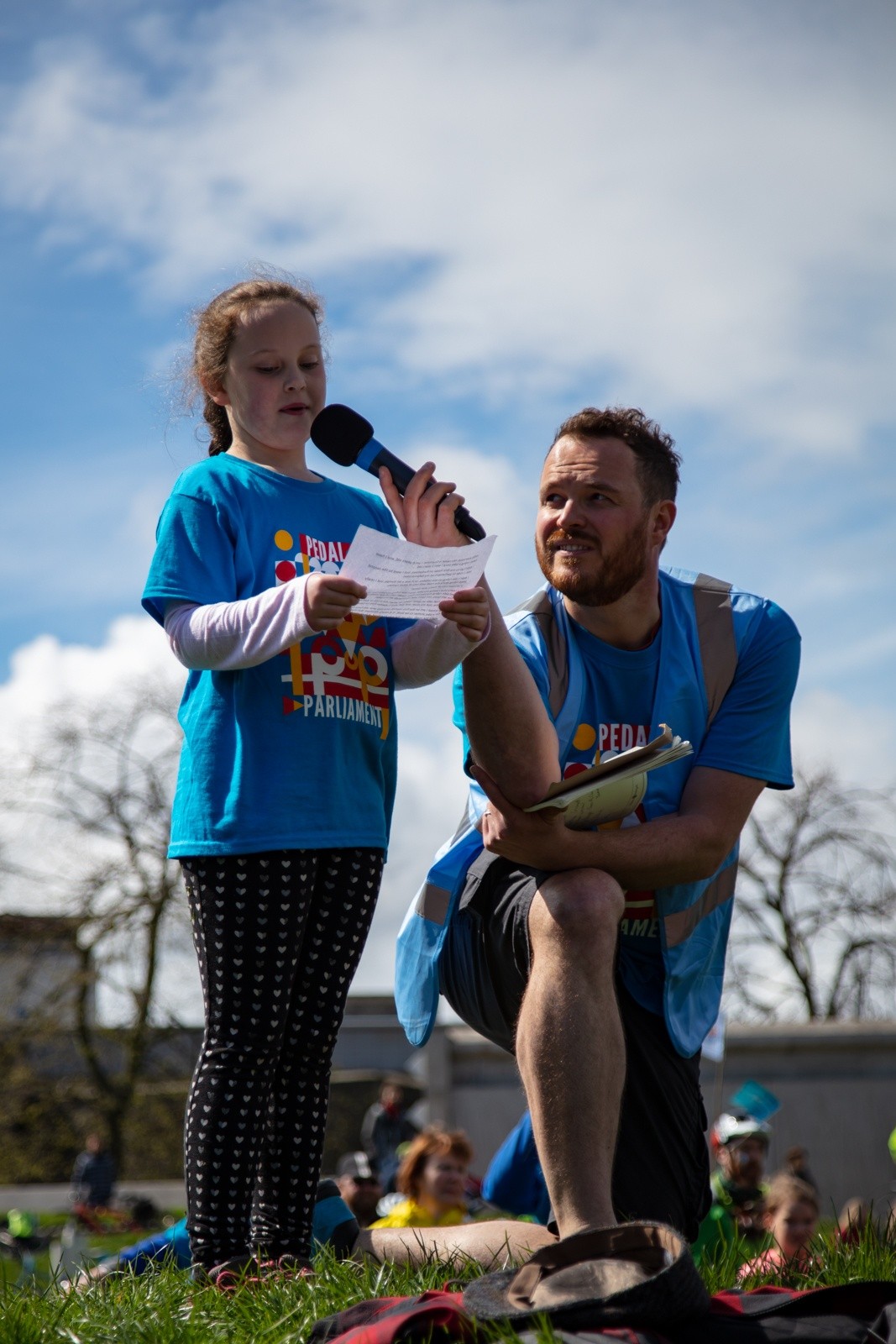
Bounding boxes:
730,1080,781,1130
701,1012,727,1063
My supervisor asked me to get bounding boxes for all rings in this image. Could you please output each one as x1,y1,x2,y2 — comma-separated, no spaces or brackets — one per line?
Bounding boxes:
483,809,490,817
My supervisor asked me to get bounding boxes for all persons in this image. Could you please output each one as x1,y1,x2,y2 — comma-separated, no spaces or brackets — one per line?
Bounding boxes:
140,285,491,1299
692,1109,896,1268
380,407,801,1243
338,1079,552,1228
738,1174,824,1284
71,1133,113,1210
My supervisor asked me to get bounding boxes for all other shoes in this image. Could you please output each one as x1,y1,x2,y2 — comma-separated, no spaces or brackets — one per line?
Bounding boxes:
193,1260,273,1296
257,1256,317,1284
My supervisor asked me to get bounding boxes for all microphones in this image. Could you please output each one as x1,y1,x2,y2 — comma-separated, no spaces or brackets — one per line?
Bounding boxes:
311,404,487,543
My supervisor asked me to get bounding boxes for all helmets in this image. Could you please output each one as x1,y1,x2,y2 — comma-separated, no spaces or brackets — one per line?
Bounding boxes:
709,1111,774,1152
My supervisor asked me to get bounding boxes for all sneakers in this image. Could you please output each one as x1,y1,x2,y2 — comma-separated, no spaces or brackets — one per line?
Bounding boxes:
313,1181,359,1260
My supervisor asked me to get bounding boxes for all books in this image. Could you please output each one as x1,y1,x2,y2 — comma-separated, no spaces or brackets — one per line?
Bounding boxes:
522,722,694,829
